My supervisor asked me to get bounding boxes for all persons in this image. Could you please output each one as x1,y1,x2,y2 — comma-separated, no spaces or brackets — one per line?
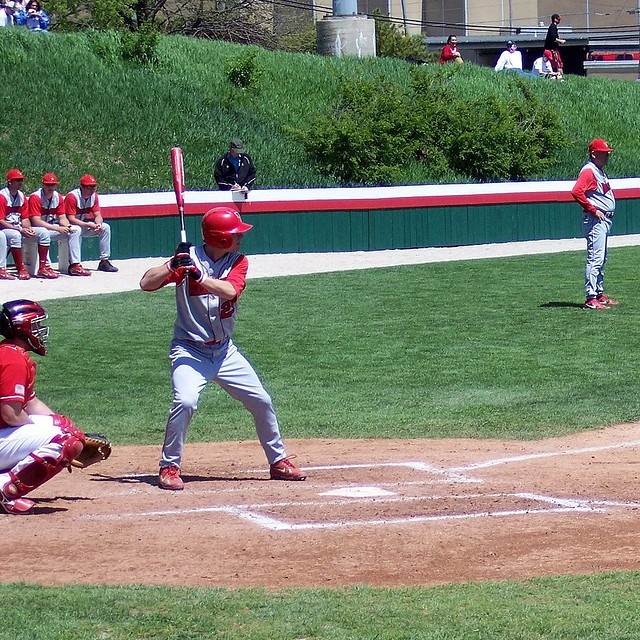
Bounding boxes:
139,208,310,491
0,2,20,26
570,139,619,310
494,40,523,71
19,0,50,31
544,14,566,75
0,300,111,516
214,139,256,191
0,169,60,280
0,231,16,280
439,34,463,64
532,50,558,78
64,173,118,276
10,0,28,25
28,173,92,279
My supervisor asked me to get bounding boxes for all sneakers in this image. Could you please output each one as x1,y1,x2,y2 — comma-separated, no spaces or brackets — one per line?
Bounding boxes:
98,260,118,271
35,268,59,279
158,466,184,490
68,263,91,276
0,268,15,280
597,294,619,305
0,498,36,515
585,298,610,309
18,268,30,280
269,455,307,481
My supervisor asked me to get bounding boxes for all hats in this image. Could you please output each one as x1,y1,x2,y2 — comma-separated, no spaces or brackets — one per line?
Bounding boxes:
231,138,246,153
4,1,16,8
551,14,564,19
506,40,516,45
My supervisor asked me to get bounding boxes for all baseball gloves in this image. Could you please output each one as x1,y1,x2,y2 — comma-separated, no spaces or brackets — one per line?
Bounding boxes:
72,433,111,469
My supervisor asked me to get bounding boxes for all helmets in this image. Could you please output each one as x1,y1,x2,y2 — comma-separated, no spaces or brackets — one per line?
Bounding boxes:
42,173,57,185
543,50,553,62
6,168,26,180
0,299,49,357
201,207,253,248
588,138,614,152
79,174,97,186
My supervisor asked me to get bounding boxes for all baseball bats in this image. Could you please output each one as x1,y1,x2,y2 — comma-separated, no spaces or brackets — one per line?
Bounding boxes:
170,147,190,276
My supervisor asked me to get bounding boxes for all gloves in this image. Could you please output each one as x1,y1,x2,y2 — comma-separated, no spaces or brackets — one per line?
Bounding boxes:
175,243,208,285
168,245,192,276
